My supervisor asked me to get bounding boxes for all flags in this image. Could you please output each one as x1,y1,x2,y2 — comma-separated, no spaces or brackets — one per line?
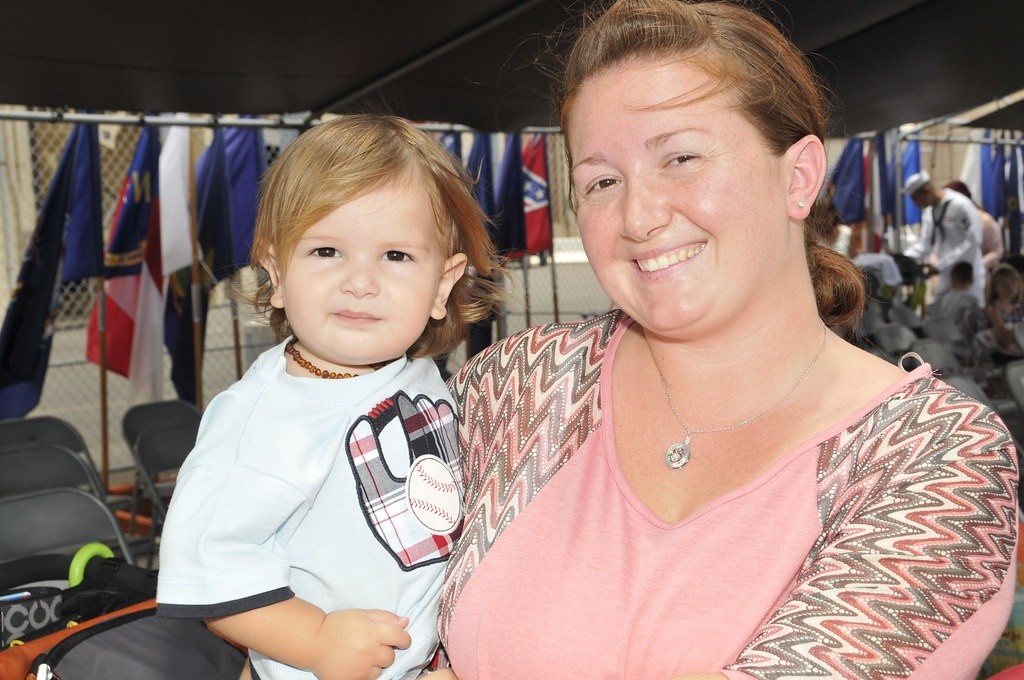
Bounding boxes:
0,122,555,417
825,124,1024,254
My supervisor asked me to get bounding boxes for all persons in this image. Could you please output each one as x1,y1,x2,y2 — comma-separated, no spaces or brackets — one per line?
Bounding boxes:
239,1,1019,680
829,172,1024,368
156,115,517,680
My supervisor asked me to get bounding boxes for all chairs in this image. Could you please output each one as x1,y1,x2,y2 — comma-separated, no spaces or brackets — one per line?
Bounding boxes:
0,401,249,680
861,306,1024,471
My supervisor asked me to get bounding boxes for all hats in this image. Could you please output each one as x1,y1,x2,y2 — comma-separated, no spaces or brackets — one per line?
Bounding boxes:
904,169,930,196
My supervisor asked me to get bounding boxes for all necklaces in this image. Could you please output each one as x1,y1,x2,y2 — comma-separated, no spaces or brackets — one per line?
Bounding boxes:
659,317,825,469
285,343,390,380
